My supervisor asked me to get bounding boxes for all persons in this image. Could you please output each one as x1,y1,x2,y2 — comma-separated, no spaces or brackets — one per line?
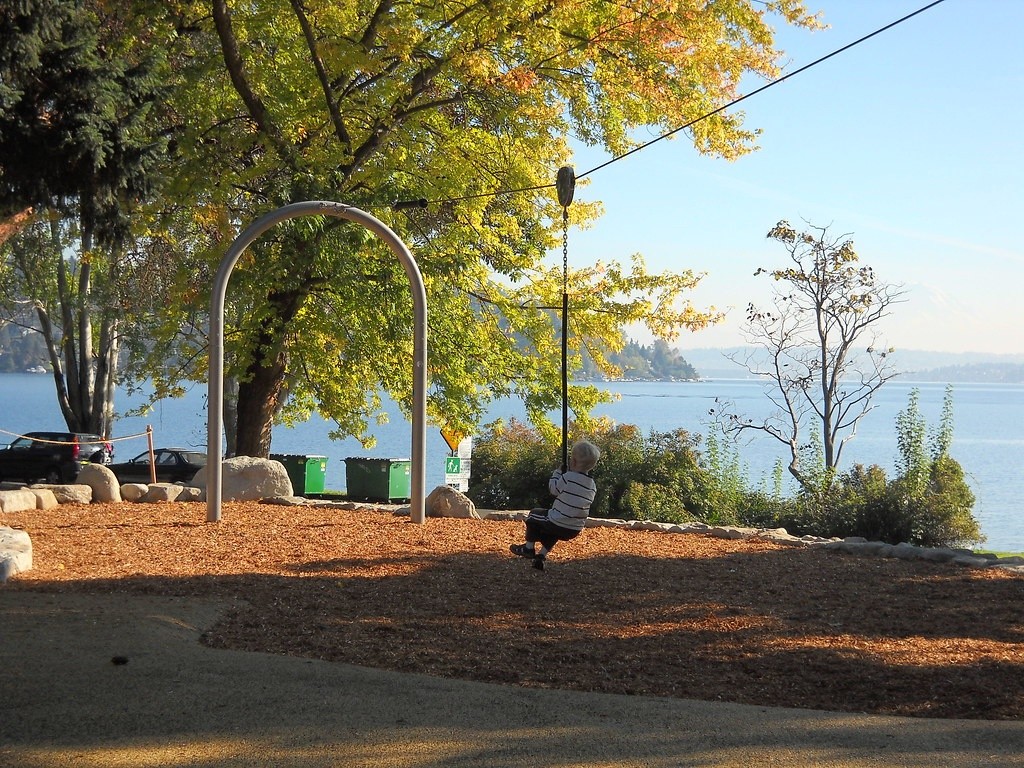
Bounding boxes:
509,441,600,570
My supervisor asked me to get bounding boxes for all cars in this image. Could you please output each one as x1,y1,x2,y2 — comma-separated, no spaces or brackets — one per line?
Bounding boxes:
109,448,208,485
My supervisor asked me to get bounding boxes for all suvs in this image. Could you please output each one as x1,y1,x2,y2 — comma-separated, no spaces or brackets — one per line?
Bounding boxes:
0,431,112,485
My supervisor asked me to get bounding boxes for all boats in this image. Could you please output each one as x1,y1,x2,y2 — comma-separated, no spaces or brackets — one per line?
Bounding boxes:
36,365,48,374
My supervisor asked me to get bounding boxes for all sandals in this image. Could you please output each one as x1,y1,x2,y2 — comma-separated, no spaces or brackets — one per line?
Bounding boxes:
510,544,535,559
532,555,546,570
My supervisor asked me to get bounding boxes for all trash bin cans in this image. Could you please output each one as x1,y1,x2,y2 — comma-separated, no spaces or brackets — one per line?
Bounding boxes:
270,453,329,499
339,456,411,504
